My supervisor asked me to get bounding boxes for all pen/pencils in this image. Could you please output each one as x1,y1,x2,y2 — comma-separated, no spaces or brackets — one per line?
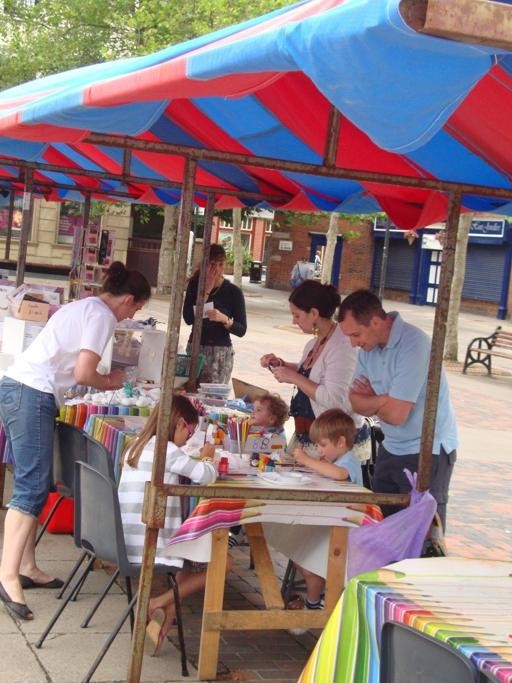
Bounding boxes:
291,440,305,471
337,504,386,529
169,498,267,541
204,434,206,444
192,397,206,416
228,415,251,459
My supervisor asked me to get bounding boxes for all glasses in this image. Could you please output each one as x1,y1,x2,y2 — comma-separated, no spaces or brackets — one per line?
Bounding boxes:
183,419,195,439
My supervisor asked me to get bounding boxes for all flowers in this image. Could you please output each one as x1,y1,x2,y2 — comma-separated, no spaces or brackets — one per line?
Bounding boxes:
435,229,446,240
404,229,420,239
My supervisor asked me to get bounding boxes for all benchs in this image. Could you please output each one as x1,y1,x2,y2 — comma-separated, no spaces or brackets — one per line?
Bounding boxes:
462,325,512,375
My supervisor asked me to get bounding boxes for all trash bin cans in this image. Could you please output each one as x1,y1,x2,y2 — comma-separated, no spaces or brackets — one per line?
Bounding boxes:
250,262,262,283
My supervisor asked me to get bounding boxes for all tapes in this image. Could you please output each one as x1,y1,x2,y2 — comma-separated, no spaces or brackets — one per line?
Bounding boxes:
269,358,284,373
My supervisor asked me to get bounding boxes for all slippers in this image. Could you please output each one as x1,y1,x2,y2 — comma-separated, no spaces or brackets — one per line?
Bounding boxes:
144,607,167,657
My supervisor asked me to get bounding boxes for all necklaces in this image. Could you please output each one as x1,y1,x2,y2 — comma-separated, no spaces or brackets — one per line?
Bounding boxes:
306,321,333,368
213,276,222,295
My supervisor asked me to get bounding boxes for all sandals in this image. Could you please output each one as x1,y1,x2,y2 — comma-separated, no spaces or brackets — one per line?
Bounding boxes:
0,581,34,620
17,573,67,588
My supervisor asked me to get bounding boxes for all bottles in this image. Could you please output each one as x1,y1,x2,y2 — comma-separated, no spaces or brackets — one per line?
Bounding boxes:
218,456,229,480
250,444,282,472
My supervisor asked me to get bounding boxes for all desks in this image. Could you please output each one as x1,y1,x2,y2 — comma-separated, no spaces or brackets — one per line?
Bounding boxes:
81,413,385,681
296,556,512,683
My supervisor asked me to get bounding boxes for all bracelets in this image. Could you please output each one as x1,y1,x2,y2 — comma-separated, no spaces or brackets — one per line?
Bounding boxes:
278,358,286,367
200,457,214,464
223,315,230,327
106,372,112,392
205,284,212,296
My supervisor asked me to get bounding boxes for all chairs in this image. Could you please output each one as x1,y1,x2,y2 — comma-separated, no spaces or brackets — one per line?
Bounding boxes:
33,459,192,683
34,421,87,547
55,433,115,628
379,620,479,683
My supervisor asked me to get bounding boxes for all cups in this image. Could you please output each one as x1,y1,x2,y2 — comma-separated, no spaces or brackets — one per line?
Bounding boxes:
230,438,243,453
122,388,161,407
137,328,166,382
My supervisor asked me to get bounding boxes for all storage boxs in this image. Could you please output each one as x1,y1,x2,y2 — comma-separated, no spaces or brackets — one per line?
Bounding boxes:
111,327,146,368
1,317,45,357
10,292,50,322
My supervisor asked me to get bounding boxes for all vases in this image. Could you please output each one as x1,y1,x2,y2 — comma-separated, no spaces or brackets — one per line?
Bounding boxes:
408,234,414,245
439,239,445,246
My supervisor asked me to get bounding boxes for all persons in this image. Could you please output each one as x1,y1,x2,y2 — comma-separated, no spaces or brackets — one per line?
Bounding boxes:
182,243,247,382
0,260,152,620
289,408,366,635
116,394,234,660
314,250,321,269
248,394,288,448
334,288,457,538
261,278,363,610
